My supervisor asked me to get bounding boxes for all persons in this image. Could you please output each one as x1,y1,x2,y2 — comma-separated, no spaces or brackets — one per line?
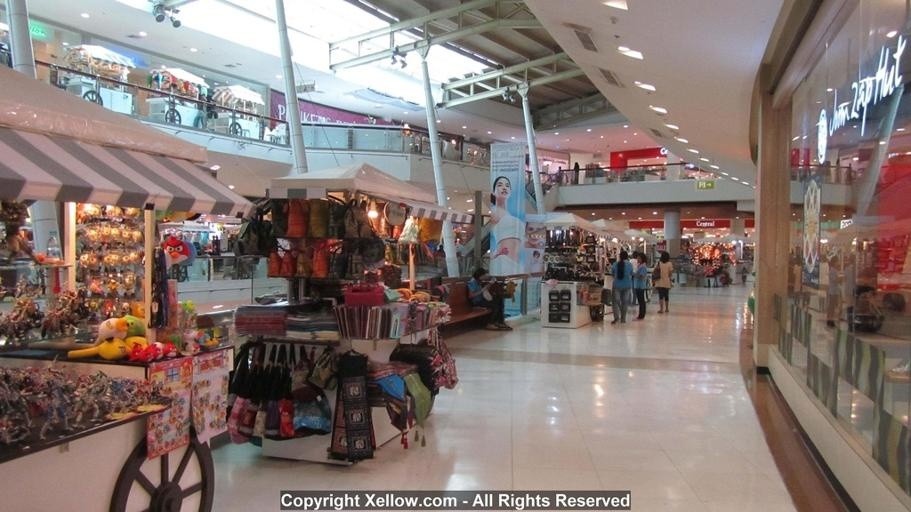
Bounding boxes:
161,236,190,265
630,251,640,304
654,252,674,313
490,176,525,275
467,267,514,330
611,250,633,324
632,252,647,321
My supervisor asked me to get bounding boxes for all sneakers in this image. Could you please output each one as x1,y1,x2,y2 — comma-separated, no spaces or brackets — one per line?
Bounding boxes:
610,318,620,324
657,310,664,313
632,316,645,321
665,310,669,312
621,319,626,323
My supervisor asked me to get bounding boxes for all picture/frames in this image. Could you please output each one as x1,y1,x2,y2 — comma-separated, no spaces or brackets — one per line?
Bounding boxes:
548,290,573,324
586,236,597,263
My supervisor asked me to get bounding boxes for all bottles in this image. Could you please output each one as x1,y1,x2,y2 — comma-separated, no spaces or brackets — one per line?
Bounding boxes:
49,232,60,259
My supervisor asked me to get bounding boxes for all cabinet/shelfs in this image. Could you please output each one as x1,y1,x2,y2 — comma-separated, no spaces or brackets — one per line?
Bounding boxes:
541,279,605,329
0,195,442,511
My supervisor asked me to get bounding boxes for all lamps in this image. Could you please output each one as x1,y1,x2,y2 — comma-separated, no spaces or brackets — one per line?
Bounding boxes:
148,0,180,29
389,49,410,70
502,87,519,104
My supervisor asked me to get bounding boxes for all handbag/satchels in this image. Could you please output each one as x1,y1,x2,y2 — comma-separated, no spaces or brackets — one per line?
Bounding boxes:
651,265,662,281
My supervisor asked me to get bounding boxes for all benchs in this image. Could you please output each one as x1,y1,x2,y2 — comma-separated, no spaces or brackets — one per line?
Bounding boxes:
430,274,492,329
